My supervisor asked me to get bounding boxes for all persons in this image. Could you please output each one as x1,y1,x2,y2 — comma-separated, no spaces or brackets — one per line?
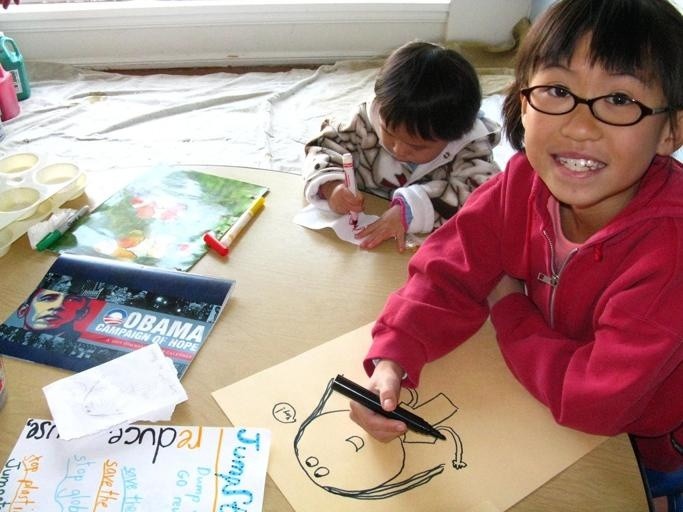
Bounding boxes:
298,40,503,257
350,1,683,512
11,291,94,332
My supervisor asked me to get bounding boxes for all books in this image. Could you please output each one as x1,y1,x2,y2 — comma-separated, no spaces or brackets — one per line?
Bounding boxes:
0,252,237,398
45,165,269,268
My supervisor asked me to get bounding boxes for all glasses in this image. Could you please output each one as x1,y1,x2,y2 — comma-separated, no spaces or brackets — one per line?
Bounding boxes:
512,84,670,127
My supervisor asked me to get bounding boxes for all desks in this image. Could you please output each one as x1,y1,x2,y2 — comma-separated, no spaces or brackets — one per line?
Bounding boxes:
0,166,655,512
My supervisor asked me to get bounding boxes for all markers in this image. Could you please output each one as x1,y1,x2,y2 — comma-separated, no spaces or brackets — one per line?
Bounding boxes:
220,196,265,248
342,152,358,226
35,205,89,252
330,374,446,440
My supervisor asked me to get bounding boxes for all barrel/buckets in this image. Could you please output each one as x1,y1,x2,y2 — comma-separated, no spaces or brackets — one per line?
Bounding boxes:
0,65,20,121
0,32,30,102
